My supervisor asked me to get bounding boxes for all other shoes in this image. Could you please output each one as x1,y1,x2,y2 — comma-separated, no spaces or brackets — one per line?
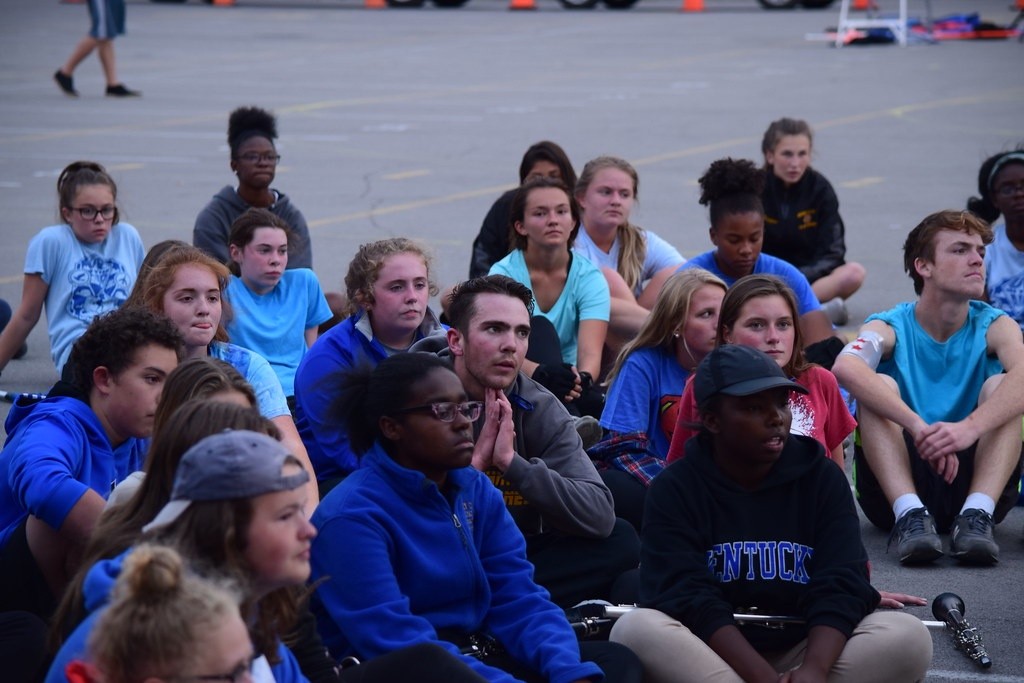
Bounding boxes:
55,70,76,96
106,84,140,97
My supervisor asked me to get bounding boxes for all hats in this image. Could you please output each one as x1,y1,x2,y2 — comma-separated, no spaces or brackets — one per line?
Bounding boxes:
141,427,309,534
694,344,809,410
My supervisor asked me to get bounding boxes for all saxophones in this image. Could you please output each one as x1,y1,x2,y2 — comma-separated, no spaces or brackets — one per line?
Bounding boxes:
932,592,992,669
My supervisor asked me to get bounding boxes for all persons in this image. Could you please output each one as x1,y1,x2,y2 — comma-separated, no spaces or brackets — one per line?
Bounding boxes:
683,159,835,350
0,311,186,683
207,208,334,423
64,543,256,683
609,344,933,683
471,142,577,278
54,402,283,643
573,158,665,350
761,118,860,326
192,107,316,274
54,0,139,97
106,357,261,509
590,264,728,539
968,151,1024,334
47,427,316,683
409,275,637,639
666,274,929,609
489,179,613,450
0,161,133,372
130,241,321,517
294,240,439,498
310,353,606,683
831,211,1024,563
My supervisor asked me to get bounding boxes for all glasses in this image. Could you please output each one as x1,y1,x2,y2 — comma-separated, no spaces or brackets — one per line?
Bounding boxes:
993,184,1023,198
161,652,254,683
237,150,280,167
399,401,482,423
69,203,116,220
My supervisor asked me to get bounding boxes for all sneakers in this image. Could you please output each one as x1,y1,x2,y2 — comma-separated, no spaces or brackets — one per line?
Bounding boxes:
949,509,1000,562
887,507,944,564
